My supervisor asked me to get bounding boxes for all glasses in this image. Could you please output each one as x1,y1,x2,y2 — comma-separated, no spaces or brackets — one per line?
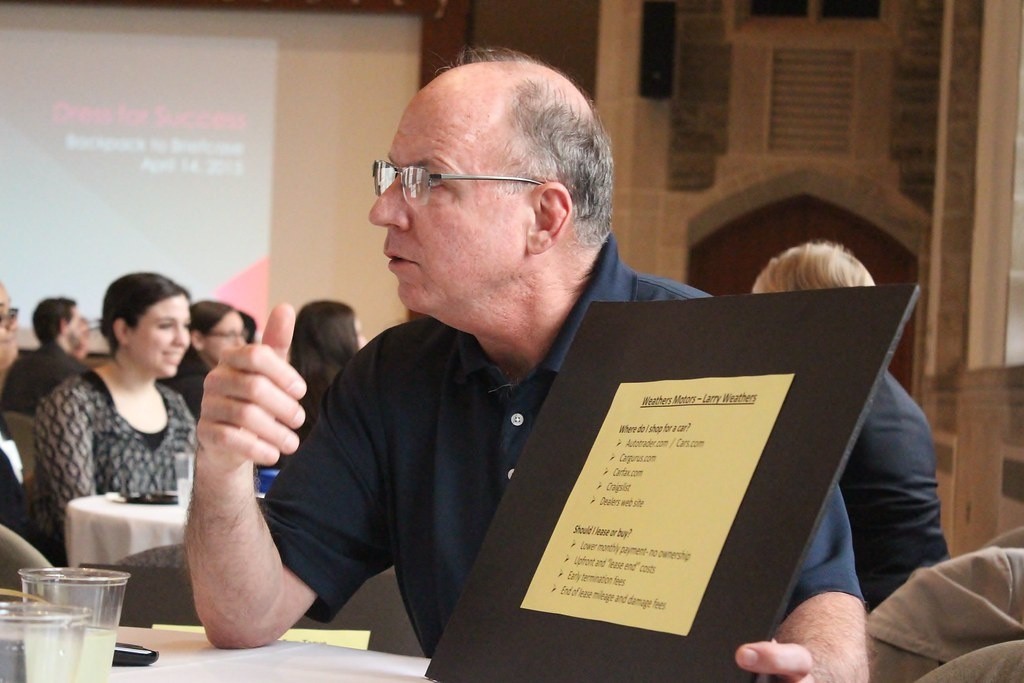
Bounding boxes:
0,307,20,328
205,329,249,341
371,160,545,209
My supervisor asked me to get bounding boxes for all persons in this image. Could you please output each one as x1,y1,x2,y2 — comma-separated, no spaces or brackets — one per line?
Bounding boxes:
0,272,368,568
185,40,871,683
867,545,1024,683
753,242,949,613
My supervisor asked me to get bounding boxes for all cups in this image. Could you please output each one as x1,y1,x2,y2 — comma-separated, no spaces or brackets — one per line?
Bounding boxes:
174,452,195,510
1,602,93,683
18,568,131,683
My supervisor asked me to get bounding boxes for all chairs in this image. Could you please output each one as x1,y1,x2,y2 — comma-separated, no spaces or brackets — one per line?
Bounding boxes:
113,542,424,659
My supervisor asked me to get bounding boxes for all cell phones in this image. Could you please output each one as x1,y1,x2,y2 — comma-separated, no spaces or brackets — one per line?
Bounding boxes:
111,642,158,666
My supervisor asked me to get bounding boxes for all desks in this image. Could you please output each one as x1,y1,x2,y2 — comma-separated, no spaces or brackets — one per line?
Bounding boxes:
0,620,431,683
63,491,187,568
